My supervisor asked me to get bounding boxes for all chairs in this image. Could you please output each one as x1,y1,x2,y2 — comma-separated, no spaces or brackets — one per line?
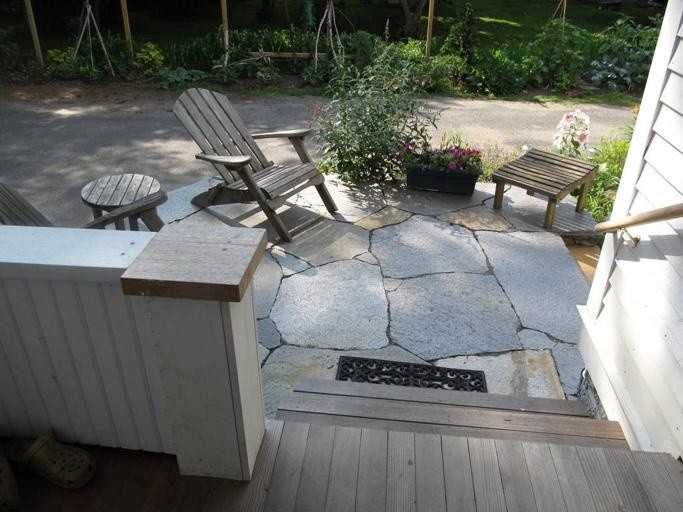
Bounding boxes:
174,87,339,244
0,178,168,234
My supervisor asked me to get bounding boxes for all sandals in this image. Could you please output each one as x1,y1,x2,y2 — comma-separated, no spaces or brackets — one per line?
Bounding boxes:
0,430,98,510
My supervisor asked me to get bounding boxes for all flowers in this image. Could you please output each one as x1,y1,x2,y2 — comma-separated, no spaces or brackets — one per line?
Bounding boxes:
404,133,485,175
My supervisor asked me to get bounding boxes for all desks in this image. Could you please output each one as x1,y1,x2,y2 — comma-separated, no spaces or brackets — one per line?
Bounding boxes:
492,147,598,230
80,173,160,233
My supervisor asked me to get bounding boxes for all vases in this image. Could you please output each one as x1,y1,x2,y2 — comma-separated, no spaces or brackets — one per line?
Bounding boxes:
406,172,480,198
